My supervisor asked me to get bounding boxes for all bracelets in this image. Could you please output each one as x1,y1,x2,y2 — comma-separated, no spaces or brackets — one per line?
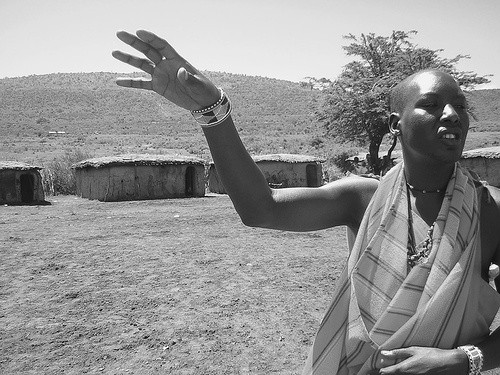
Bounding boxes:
190,86,233,128
456,342,484,375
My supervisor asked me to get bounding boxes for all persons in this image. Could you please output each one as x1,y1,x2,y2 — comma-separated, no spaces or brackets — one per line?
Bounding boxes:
111,27,499,375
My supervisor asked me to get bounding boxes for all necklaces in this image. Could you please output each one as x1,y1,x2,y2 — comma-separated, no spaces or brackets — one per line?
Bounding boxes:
405,180,446,195
